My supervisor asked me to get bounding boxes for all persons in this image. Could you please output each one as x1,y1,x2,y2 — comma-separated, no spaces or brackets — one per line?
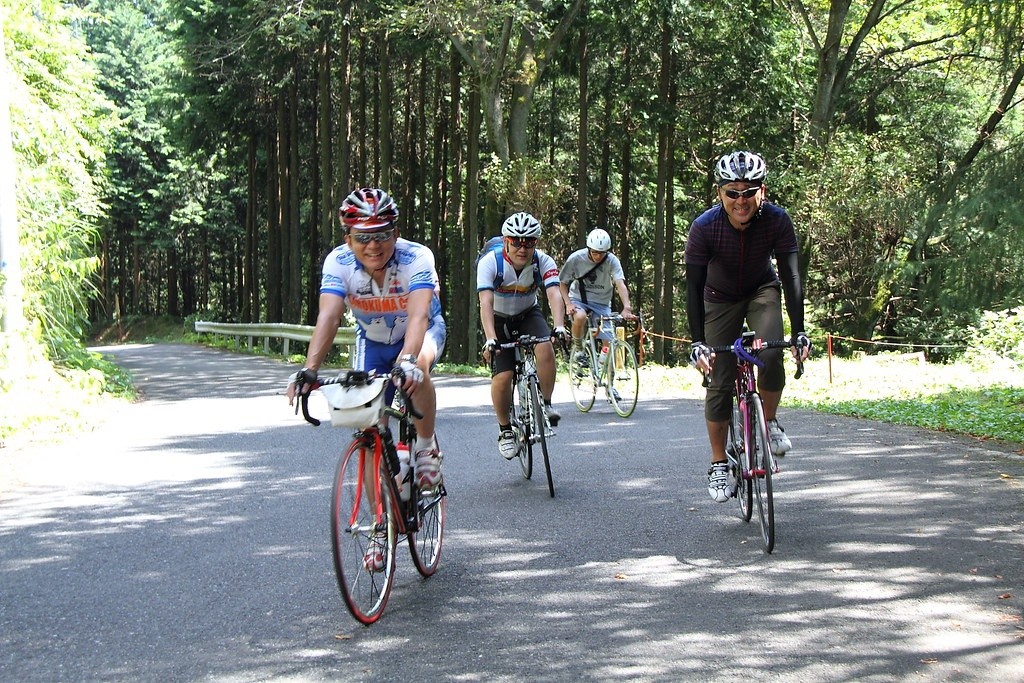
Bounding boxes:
559,229,637,400
477,212,570,460
287,188,446,572
685,152,812,502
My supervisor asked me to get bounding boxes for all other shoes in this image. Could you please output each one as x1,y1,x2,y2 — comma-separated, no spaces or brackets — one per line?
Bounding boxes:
605,388,622,401
575,350,589,366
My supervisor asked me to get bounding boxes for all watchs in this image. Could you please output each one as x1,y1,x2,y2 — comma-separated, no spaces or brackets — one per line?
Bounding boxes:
399,354,417,364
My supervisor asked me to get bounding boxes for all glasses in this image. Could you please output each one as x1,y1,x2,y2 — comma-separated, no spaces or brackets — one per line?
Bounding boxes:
507,237,538,249
348,228,395,244
726,185,761,199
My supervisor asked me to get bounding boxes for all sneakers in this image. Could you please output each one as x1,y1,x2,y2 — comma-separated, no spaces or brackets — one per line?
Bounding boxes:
362,529,387,573
766,419,792,455
498,424,516,459
544,405,561,427
415,448,444,489
707,461,731,503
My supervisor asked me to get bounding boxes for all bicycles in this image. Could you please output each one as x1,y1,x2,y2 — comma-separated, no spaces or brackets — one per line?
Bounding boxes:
701,325,805,552
569,309,643,418
294,367,449,625
488,330,570,498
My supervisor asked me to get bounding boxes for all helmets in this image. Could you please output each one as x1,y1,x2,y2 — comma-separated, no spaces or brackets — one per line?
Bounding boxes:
502,212,542,240
339,188,399,231
587,229,611,251
714,150,766,188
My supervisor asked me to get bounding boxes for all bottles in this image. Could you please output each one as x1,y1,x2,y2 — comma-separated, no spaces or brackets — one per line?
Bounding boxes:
395,441,410,501
395,473,403,493
519,377,526,407
599,346,608,365
739,392,746,441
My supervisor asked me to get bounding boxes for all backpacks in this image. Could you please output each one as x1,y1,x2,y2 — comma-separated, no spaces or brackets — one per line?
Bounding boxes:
475,236,542,290
